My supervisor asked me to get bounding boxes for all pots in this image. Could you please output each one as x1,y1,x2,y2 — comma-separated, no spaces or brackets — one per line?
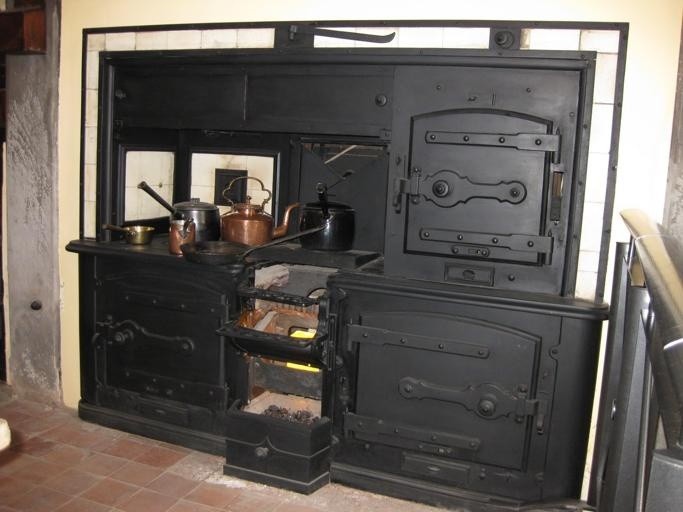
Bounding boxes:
179,223,328,265
136,180,220,243
102,221,156,245
299,180,358,251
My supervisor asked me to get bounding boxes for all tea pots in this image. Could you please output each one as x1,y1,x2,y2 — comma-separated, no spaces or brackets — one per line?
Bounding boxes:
220,176,300,248
